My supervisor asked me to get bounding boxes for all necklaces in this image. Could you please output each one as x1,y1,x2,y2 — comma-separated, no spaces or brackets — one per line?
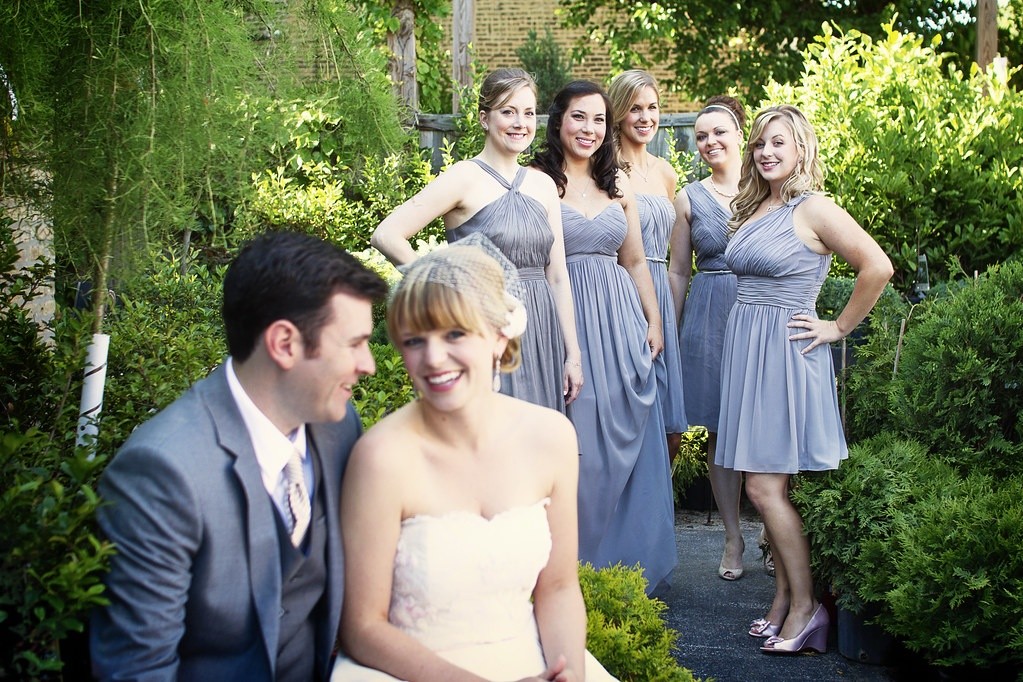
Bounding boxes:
624,159,650,183
570,177,591,197
766,197,779,212
710,173,740,198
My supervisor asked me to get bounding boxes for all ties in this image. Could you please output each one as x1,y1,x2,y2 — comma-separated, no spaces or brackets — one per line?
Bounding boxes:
283,448,312,549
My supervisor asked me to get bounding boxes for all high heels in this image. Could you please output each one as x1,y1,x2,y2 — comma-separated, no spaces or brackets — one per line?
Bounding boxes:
749,601,793,637
759,528,778,576
760,602,830,654
718,536,746,580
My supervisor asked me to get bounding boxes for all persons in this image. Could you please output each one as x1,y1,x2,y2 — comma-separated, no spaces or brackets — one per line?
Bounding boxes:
712,103,895,656
606,68,689,466
92,224,388,681
529,80,678,598
666,97,776,582
332,232,619,681
371,67,585,456
